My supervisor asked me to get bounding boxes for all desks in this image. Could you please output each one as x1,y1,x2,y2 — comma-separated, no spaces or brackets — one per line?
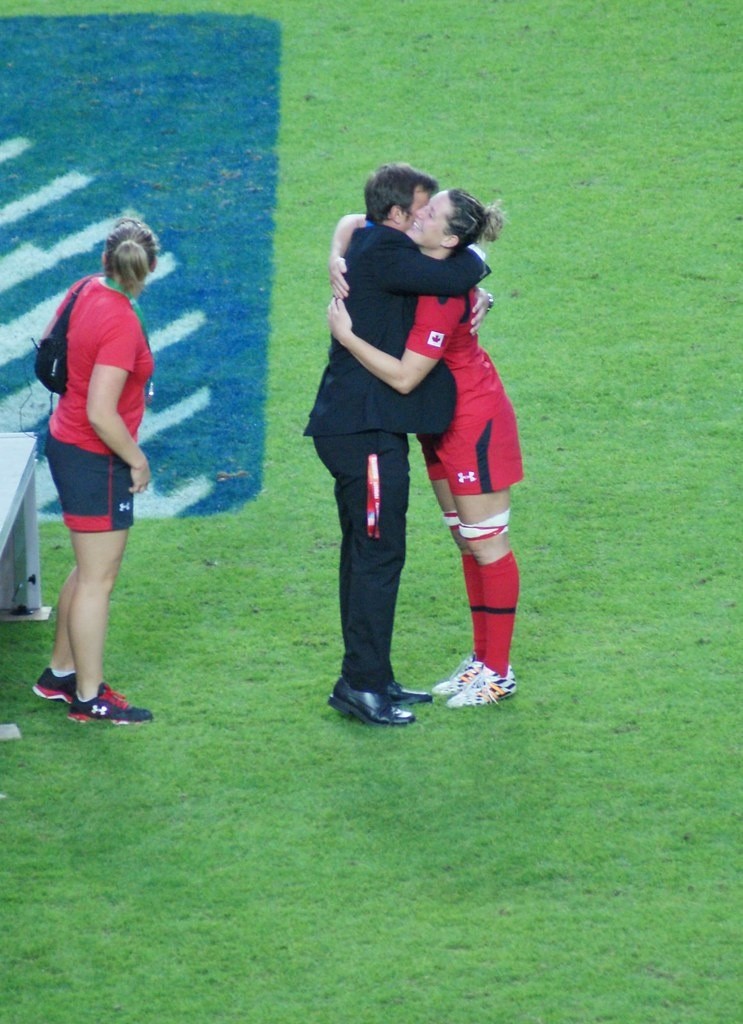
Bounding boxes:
0,431,43,613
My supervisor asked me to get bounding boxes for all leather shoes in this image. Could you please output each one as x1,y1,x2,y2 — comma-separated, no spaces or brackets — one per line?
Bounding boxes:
326,676,417,725
385,679,431,705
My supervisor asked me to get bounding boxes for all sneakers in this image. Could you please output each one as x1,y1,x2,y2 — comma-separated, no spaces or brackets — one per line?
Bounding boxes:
446,660,518,709
66,683,154,725
433,652,483,694
30,667,80,706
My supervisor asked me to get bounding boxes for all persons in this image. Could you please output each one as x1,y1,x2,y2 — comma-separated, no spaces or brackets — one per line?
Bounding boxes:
302,161,491,728
325,188,526,709
27,217,163,724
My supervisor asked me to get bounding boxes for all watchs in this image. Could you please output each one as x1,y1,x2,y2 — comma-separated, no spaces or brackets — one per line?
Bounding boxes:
486,293,493,314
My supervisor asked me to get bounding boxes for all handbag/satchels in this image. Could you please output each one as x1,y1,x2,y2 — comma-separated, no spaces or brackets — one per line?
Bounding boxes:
32,277,101,395
